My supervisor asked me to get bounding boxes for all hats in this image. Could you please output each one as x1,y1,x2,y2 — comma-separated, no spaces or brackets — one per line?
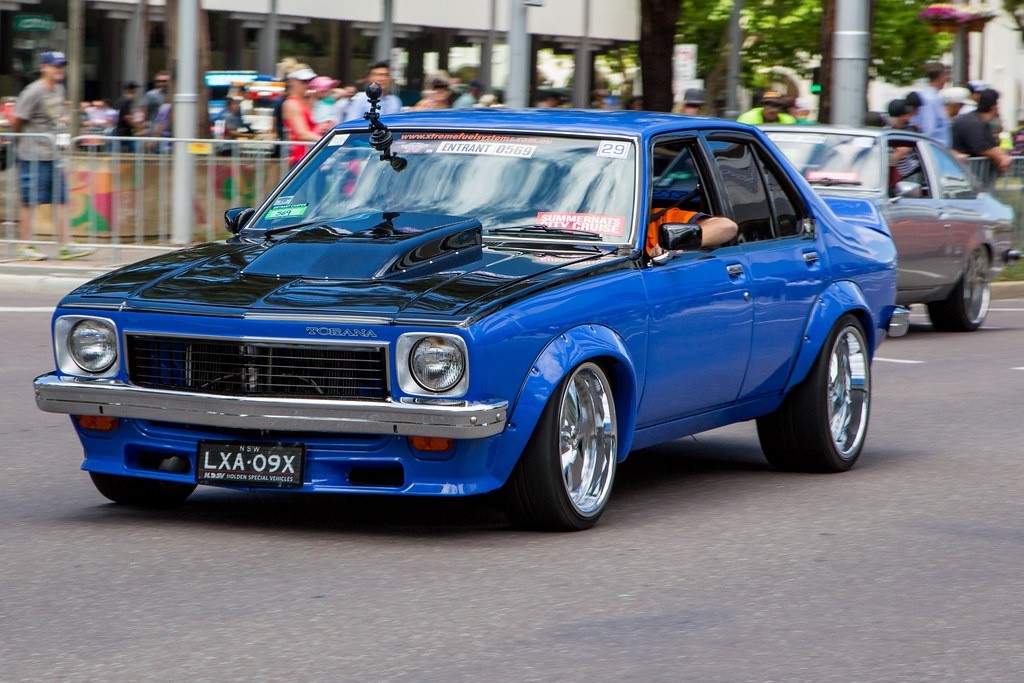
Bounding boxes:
940,87,977,108
123,81,141,89
308,76,340,91
40,51,68,66
758,90,786,105
287,68,318,81
684,89,706,104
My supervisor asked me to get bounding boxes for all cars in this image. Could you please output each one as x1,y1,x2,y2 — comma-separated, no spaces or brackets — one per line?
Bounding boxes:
760,123,1024,333
203,68,272,123
34,81,910,535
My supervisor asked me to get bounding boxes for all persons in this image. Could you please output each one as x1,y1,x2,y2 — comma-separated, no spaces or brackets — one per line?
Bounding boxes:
620,83,642,108
862,93,923,183
738,91,810,124
413,78,482,108
1010,119,1024,156
533,92,562,106
910,61,1012,171
683,89,706,114
647,208,738,254
59,72,170,152
216,59,401,164
12,51,96,260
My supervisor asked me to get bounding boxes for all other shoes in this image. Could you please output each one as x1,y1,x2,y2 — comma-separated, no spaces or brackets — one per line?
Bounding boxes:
57,242,97,259
16,247,48,261
